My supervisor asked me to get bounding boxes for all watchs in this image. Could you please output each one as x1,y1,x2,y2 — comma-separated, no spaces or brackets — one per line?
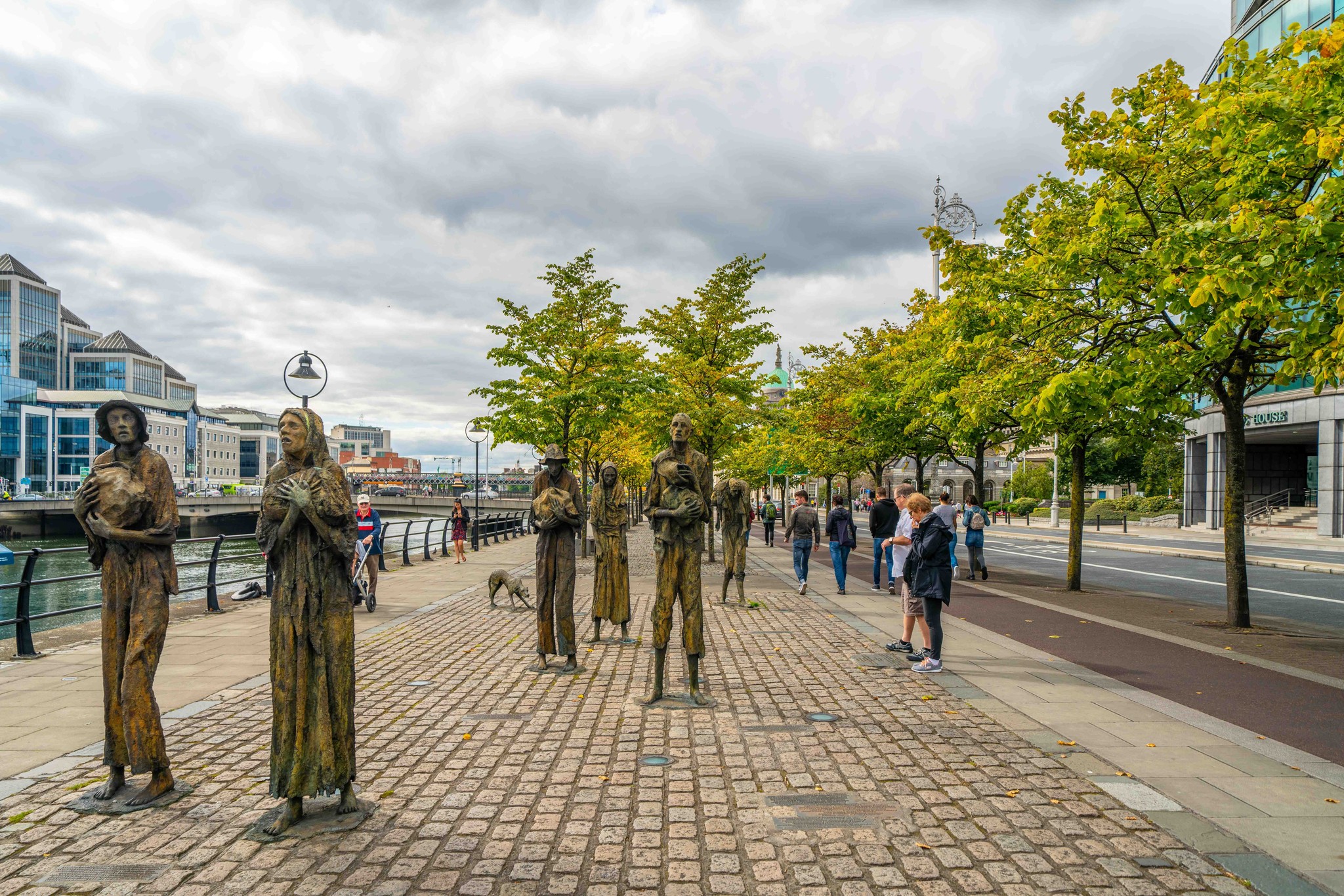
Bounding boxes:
369,535,374,540
888,537,894,544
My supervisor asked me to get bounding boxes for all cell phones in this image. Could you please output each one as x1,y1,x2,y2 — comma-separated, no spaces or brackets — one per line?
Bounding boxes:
455,512,458,515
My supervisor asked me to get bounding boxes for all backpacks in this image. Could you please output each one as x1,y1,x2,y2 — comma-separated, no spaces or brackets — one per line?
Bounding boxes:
764,502,775,520
968,509,984,530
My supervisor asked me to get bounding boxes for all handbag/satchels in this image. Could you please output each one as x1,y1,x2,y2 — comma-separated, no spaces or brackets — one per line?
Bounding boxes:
466,521,472,528
823,504,826,507
837,511,858,546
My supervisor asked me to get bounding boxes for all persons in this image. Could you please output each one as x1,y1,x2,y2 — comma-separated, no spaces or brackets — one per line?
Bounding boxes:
255,407,359,836
529,451,631,672
811,498,816,509
72,399,181,807
869,483,954,673
219,484,224,496
642,412,712,706
925,491,960,581
962,494,991,580
0,491,10,500
760,494,777,547
710,477,754,609
950,500,970,519
854,497,875,513
423,483,433,498
824,497,826,509
825,494,857,595
450,497,470,564
354,494,381,608
751,497,757,505
745,511,755,547
488,486,493,500
784,490,821,595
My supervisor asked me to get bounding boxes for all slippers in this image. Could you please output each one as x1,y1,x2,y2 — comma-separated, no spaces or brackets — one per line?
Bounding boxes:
454,561,460,564
462,555,466,562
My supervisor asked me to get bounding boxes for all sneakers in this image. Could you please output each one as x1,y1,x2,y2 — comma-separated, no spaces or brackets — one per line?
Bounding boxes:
913,656,943,669
911,657,941,673
906,647,931,662
886,639,913,653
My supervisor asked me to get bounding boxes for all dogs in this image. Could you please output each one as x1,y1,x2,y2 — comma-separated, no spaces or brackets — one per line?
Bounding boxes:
488,569,535,611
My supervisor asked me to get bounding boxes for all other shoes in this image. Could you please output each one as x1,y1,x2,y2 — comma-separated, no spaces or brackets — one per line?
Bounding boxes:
953,566,988,580
889,585,896,594
764,540,774,547
837,585,845,595
745,539,748,547
353,601,362,606
799,581,807,595
871,585,880,591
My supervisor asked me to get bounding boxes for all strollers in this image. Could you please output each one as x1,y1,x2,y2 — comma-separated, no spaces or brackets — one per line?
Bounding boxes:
351,540,376,612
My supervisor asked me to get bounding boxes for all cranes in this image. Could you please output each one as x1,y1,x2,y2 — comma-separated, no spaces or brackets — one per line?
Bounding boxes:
433,456,493,473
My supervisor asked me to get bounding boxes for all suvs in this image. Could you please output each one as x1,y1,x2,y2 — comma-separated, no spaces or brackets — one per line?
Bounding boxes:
374,486,406,497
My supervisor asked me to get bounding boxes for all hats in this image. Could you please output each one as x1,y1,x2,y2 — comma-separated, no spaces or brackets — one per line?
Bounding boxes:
357,494,370,504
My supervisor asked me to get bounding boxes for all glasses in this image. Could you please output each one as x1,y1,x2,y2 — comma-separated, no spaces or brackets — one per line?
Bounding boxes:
454,499,460,503
893,496,906,502
359,502,369,505
908,510,914,516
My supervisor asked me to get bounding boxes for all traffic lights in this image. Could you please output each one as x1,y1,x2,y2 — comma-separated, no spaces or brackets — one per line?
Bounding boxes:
49,481,52,486
44,481,48,487
2,477,4,484
70,481,73,486
7,479,9,487
4,479,8,485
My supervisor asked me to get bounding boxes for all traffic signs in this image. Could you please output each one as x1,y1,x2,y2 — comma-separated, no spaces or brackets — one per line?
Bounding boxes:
81,478,86,483
80,472,89,477
80,467,90,472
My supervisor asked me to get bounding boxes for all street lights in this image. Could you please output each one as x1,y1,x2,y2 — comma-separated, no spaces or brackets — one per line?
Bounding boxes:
282,350,328,406
204,408,220,488
532,445,545,472
258,453,266,487
485,407,495,499
464,417,489,551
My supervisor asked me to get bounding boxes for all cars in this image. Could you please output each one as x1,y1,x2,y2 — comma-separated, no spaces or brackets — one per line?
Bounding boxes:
187,488,220,497
460,488,498,500
12,494,45,501
250,487,264,496
174,489,186,498
